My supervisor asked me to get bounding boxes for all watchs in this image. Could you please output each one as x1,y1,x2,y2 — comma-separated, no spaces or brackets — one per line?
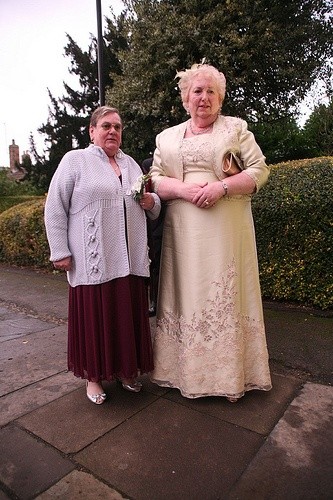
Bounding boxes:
220,179,229,196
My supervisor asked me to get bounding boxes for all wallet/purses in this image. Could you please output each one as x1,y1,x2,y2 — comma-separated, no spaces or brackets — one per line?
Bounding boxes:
222,152,245,175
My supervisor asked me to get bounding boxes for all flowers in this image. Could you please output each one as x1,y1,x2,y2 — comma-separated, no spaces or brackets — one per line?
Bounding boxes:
127,174,152,201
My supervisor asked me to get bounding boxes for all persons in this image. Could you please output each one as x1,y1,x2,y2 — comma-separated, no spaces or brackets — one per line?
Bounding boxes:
44,106,161,405
148,64,272,403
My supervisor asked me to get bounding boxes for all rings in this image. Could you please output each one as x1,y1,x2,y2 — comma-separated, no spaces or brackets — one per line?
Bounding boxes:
204,200,209,205
143,203,145,206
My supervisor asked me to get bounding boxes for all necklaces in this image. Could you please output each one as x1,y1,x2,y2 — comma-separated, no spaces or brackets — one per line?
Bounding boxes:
189,120,214,135
115,165,119,171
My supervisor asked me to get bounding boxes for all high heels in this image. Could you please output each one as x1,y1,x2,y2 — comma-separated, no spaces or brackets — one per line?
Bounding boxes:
116,375,142,392
86,379,106,404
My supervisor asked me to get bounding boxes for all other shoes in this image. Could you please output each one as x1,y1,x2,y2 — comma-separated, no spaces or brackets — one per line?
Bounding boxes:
149,306,156,317
